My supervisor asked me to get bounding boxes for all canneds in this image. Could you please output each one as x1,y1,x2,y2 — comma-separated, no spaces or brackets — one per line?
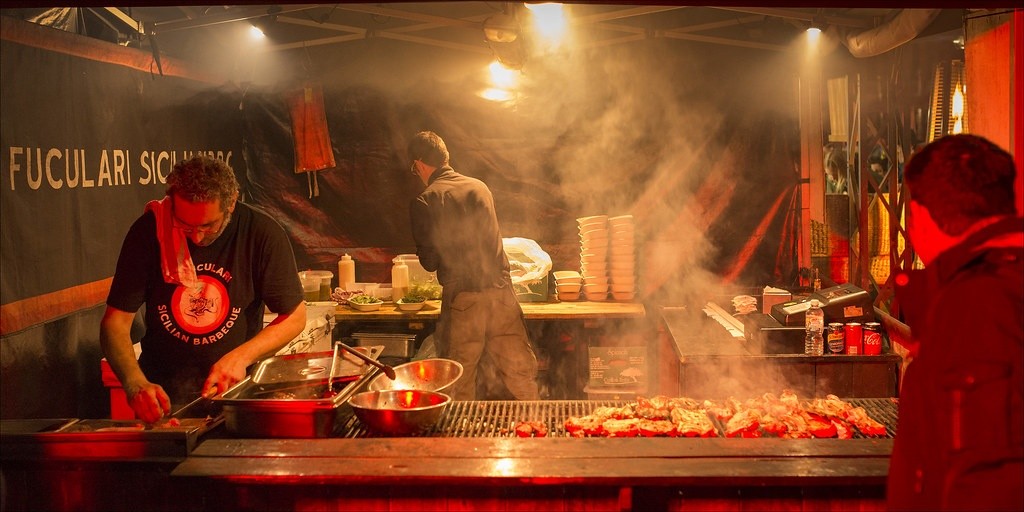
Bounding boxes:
845,322,863,356
862,322,882,355
826,323,845,354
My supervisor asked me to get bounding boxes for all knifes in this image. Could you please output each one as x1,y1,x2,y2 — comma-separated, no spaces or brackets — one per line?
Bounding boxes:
171,386,217,416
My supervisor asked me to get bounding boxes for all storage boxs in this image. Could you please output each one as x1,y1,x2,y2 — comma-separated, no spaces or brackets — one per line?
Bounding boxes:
584,335,648,398
395,253,443,298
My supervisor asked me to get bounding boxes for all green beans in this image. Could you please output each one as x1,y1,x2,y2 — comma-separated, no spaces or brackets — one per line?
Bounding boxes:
351,295,382,304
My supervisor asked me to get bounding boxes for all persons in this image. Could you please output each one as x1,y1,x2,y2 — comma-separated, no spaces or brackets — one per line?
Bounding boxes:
100,156,307,423
882,134,1024,512
408,131,541,400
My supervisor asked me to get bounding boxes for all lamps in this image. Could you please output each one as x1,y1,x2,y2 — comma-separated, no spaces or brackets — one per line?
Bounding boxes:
252,6,282,36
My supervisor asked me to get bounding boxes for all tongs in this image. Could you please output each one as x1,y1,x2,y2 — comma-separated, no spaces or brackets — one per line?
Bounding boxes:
329,341,396,391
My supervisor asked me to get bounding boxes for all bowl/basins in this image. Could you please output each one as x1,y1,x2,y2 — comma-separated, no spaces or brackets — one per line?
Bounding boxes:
366,358,463,396
348,389,451,435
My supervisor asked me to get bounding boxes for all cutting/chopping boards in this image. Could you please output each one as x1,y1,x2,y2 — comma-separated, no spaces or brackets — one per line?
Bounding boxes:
0,418,80,434
56,419,210,434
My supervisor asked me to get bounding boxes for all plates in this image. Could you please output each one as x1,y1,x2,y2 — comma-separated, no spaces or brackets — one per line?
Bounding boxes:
347,296,384,311
553,270,582,301
397,296,427,310
608,214,637,301
575,215,608,301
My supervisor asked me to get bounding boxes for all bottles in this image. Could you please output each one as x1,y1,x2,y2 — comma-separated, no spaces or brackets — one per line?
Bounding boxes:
339,253,354,293
805,299,824,357
391,257,408,303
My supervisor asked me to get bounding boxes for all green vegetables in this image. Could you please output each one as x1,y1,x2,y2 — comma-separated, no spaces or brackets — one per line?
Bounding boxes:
401,292,426,304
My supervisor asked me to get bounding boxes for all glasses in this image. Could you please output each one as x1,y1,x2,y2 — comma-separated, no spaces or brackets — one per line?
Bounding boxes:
411,157,423,176
170,206,227,235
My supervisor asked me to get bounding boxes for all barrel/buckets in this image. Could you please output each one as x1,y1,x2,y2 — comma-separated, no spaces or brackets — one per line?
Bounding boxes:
304,270,333,301
300,275,322,301
